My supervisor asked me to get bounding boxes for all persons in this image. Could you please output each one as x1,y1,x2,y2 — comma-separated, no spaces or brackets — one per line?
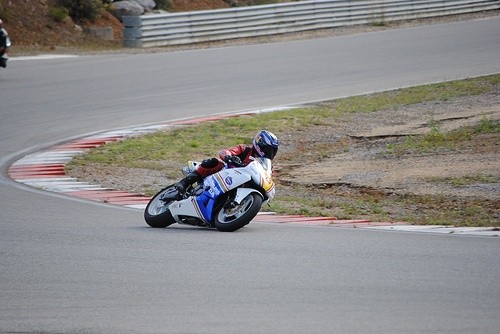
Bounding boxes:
174,129,280,193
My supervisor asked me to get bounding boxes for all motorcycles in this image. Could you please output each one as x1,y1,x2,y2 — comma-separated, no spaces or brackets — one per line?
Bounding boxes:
142,156,276,233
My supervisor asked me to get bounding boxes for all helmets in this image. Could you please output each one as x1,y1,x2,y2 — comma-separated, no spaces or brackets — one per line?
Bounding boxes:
253,130,279,159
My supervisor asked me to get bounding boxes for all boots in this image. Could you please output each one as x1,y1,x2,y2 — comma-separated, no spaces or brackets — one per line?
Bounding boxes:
173,170,201,193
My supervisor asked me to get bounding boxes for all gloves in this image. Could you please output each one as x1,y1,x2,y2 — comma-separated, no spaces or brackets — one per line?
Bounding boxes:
225,155,242,166
